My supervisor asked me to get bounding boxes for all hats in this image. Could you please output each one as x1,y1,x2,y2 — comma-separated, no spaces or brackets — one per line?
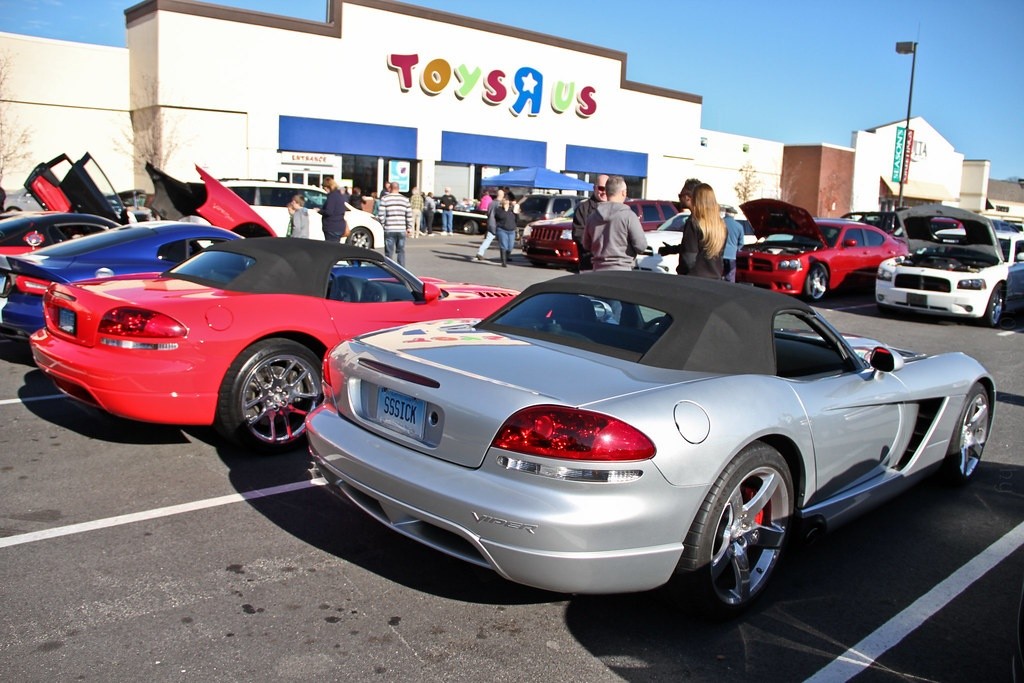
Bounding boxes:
726,207,737,214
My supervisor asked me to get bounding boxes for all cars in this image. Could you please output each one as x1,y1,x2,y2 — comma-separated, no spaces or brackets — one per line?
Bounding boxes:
634,210,766,276
876,205,1024,330
841,211,1024,238
735,198,908,301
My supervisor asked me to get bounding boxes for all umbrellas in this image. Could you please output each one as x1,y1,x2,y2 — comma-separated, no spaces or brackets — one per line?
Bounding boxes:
482,167,596,191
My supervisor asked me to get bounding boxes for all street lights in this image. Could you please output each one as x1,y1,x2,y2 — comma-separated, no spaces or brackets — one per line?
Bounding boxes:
895,40,919,206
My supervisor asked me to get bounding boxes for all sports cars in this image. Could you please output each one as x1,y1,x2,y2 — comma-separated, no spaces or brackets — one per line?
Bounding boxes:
303,270,996,627
0,151,279,366
27,236,526,461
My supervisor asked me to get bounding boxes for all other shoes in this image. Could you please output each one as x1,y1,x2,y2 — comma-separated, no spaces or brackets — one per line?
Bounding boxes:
448,232,453,235
476,254,483,260
428,233,434,236
441,231,447,236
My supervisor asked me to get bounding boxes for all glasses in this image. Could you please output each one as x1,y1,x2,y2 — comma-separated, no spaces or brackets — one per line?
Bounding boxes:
678,193,686,198
595,184,605,191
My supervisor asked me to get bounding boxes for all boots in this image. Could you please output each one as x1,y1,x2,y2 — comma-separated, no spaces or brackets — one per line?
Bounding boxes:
507,251,512,261
501,251,506,267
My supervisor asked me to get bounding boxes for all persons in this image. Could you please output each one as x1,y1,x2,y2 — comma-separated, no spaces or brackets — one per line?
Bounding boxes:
504,187,516,200
314,176,346,243
676,183,728,280
658,178,702,256
410,187,435,238
286,194,309,239
0,173,7,213
440,187,457,235
340,186,367,210
572,174,610,274
476,190,504,260
584,175,647,272
480,190,492,214
494,197,521,267
721,207,744,283
378,182,412,270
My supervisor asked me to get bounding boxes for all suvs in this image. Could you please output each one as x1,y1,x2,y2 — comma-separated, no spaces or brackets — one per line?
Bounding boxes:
521,199,678,274
185,176,385,252
514,194,591,226
